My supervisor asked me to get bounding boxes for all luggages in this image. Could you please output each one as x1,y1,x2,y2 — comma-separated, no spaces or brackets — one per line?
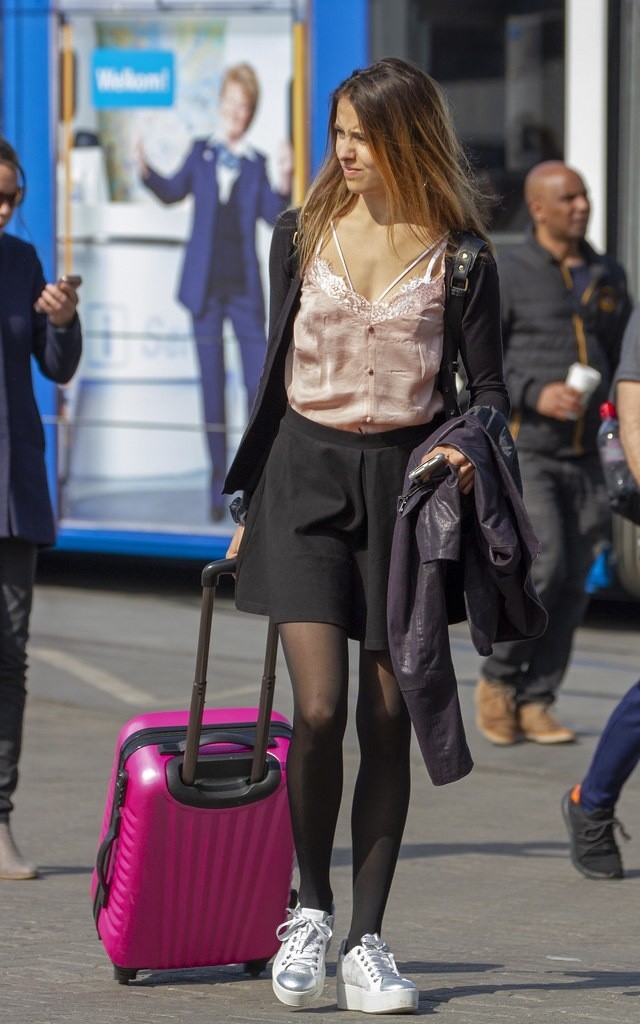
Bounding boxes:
90,557,297,987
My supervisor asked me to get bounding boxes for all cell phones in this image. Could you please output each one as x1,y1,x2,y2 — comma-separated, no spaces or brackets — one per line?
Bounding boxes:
34,275,81,312
408,453,446,484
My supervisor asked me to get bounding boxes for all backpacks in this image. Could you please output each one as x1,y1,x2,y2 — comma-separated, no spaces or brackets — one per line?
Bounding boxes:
603,365,640,526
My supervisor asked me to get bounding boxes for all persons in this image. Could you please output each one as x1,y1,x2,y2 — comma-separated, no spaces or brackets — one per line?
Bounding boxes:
559,302,640,881
223,56,546,1013
475,158,632,744
132,62,295,528
0,139,83,879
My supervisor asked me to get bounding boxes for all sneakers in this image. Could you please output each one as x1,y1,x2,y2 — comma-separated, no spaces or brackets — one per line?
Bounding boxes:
519,700,574,744
473,677,522,746
560,786,632,878
336,932,419,1014
272,903,335,1008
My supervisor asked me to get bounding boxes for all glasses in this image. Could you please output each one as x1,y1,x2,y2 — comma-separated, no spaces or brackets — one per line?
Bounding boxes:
0,186,24,208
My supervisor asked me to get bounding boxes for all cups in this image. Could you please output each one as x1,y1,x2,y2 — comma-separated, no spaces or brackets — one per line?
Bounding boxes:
560,362,602,422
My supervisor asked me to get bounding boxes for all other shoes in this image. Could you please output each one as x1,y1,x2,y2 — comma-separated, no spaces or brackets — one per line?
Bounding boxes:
0,820,37,880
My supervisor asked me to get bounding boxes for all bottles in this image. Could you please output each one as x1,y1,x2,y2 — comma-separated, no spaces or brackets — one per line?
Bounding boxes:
597,402,638,500
